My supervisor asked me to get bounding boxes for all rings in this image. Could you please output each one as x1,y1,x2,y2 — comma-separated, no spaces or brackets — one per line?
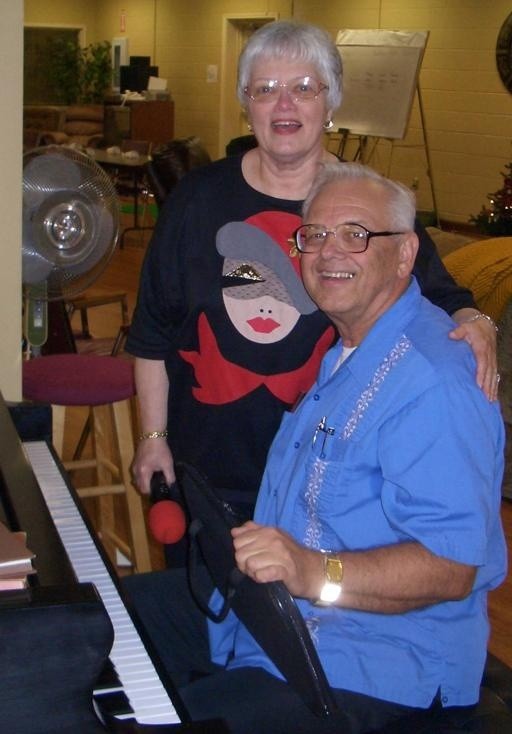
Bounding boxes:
496,374,501,383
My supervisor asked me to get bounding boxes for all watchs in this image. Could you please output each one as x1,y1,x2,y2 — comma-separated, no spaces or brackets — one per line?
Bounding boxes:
315,549,345,608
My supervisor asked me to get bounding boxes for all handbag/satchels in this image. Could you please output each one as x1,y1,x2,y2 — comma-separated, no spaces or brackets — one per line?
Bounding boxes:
178,459,340,727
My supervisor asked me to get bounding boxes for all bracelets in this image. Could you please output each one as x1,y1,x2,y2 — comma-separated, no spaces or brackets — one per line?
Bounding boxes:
141,431,169,440
466,313,500,334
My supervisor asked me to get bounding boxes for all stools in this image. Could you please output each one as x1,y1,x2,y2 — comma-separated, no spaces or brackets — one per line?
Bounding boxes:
24,358,153,574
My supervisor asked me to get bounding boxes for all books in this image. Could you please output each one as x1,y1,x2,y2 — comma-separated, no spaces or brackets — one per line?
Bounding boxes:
0,521,38,589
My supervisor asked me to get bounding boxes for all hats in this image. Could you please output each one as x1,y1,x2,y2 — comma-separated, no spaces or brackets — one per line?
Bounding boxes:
215,211,319,315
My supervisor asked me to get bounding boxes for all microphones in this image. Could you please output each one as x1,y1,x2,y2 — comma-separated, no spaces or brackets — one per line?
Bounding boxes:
148,471,187,544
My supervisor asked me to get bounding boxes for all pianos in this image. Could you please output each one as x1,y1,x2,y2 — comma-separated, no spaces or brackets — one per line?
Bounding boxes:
2,394,187,732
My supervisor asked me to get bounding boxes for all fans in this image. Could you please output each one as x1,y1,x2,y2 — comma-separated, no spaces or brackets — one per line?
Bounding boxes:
24,144,122,357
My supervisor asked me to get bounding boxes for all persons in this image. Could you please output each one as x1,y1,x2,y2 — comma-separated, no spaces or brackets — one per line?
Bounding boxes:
128,18,501,572
119,161,505,722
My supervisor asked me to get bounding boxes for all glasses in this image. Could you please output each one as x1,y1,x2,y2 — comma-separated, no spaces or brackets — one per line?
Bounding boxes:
244,75,327,104
293,223,405,254
